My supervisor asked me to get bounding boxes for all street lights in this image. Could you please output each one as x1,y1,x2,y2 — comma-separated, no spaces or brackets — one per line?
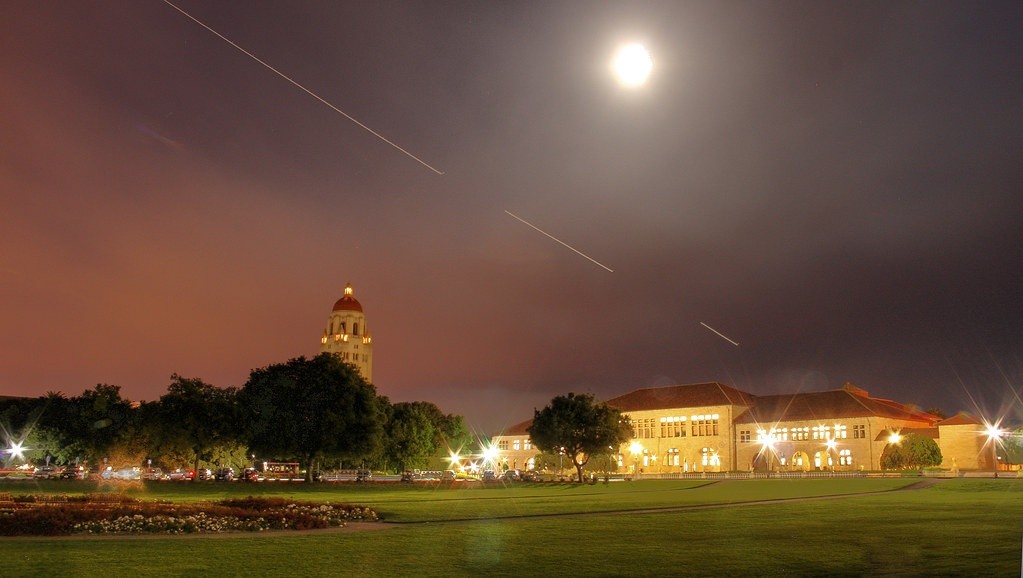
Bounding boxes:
989,427,999,478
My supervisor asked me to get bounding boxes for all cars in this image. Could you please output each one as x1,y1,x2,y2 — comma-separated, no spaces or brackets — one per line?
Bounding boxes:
523,471,538,483
239,468,260,482
141,467,164,481
191,468,211,481
357,471,372,482
503,469,521,481
60,466,84,481
168,470,186,481
33,465,62,479
402,473,415,481
483,471,497,482
215,466,234,482
440,470,456,484
